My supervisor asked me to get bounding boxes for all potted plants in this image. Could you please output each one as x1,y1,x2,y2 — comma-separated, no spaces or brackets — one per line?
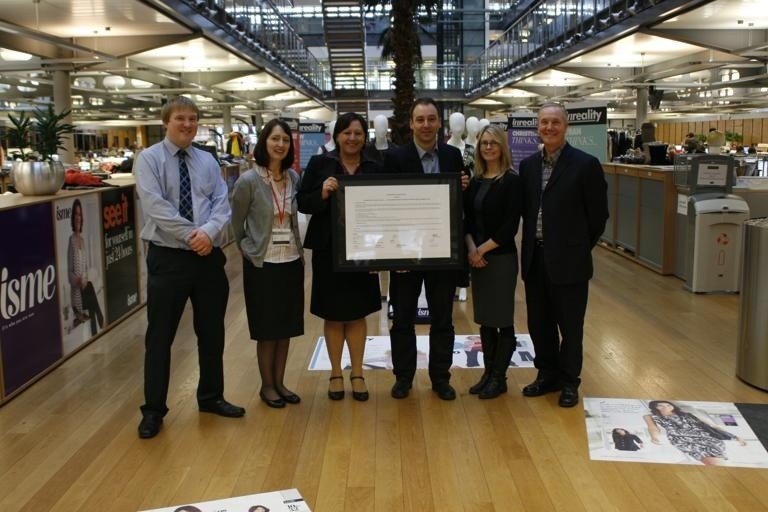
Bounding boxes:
0,99,76,198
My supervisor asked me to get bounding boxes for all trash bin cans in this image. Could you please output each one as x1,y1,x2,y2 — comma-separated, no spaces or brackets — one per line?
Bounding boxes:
736,216,768,392
683,192,750,293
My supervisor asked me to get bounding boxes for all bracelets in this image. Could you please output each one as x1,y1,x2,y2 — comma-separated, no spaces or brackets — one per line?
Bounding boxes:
475,248,481,256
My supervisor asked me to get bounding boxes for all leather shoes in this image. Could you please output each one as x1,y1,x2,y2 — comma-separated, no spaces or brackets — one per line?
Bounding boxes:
432,383,456,400
199,400,245,418
559,386,578,407
391,382,412,399
350,376,368,401
523,377,562,396
328,376,344,400
260,390,285,408
138,414,163,438
282,394,300,403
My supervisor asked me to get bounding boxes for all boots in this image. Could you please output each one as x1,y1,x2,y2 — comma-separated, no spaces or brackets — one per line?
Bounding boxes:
469,327,517,399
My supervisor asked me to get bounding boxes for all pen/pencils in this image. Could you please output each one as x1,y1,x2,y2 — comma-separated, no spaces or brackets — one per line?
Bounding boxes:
66,187,95,190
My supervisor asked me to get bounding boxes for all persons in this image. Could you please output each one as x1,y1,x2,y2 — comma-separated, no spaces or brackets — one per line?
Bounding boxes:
66,198,89,328
512,336,534,362
366,116,400,162
249,505,269,512
316,119,338,155
461,124,523,397
667,128,758,166
132,97,245,441
443,111,491,175
455,335,518,367
612,428,642,451
74,146,128,173
175,506,202,512
231,118,306,408
380,96,471,401
295,111,382,402
517,102,611,405
644,400,737,467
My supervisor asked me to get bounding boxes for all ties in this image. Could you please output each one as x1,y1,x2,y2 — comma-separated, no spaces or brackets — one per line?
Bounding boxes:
177,149,193,222
